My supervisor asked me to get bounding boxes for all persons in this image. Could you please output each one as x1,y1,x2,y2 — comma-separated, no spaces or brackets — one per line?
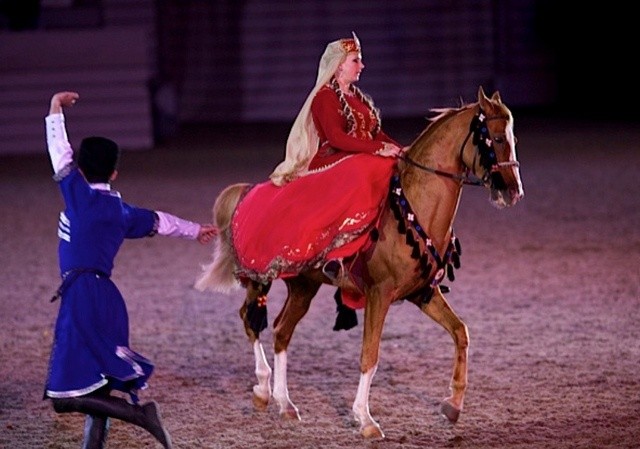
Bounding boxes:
230,7,415,331
41,90,220,448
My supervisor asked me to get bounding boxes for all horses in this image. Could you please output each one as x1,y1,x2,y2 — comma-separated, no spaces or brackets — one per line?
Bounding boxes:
193,85,524,443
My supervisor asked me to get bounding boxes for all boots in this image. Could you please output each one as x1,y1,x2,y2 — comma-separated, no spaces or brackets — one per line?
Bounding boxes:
53,394,173,448
